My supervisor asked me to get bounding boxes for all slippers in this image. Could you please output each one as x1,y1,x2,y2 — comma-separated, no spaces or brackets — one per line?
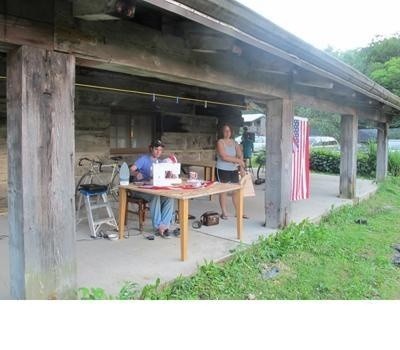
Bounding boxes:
235,214,250,219
220,215,230,220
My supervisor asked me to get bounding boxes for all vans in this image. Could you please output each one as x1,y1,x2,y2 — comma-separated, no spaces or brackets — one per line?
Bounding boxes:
310,136,340,151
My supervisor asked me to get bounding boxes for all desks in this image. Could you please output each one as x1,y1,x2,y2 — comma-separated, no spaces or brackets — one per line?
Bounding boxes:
116,181,245,258
182,161,215,181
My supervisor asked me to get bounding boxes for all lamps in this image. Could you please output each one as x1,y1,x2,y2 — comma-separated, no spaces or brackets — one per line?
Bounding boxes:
189,34,241,57
256,62,297,75
72,0,136,20
297,76,333,88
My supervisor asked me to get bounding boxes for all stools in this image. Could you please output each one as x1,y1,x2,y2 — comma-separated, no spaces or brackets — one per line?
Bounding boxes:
76,183,119,237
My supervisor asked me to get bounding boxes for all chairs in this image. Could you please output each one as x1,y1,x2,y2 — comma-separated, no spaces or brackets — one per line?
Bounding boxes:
124,164,150,232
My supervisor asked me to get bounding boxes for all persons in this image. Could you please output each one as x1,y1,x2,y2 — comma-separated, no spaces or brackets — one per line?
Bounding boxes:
241,136,253,169
216,123,247,219
241,126,253,142
129,137,174,236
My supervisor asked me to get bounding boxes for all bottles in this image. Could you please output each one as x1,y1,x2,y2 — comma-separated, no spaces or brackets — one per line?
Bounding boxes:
119,161,130,185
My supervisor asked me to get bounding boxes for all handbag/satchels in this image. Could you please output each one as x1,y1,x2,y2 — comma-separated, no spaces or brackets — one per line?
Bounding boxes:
80,183,108,192
202,210,219,226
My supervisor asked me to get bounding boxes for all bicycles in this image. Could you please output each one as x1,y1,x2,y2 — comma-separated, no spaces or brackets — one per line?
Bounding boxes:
75,157,122,210
257,149,266,181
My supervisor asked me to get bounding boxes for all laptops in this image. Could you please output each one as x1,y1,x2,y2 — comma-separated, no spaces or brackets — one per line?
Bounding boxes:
139,162,181,187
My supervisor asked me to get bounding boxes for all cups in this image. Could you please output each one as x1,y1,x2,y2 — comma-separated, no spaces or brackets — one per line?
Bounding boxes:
188,172,198,180
192,180,201,187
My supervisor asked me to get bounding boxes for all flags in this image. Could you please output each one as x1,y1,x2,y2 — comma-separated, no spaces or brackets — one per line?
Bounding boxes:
292,117,309,201
